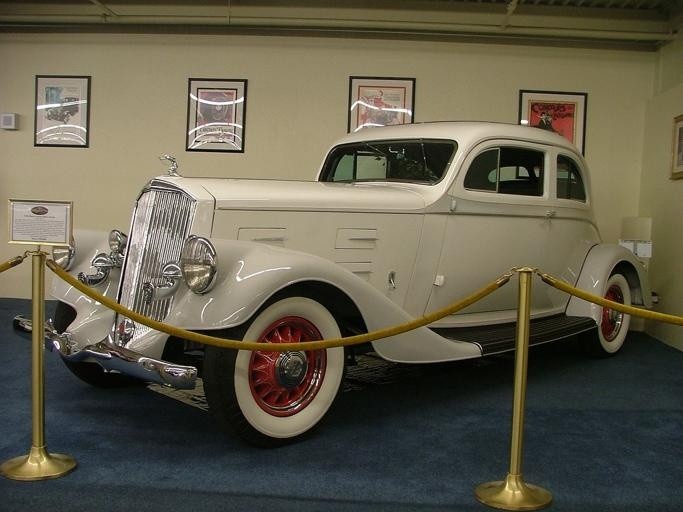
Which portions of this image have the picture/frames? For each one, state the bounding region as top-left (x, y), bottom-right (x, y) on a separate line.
top-left (517, 89), bottom-right (588, 158)
top-left (185, 78), bottom-right (248, 153)
top-left (346, 75), bottom-right (416, 135)
top-left (668, 110), bottom-right (683, 181)
top-left (33, 75), bottom-right (91, 149)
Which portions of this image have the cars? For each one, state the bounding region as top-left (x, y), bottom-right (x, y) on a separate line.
top-left (47, 98), bottom-right (79, 124)
top-left (14, 120), bottom-right (659, 449)
